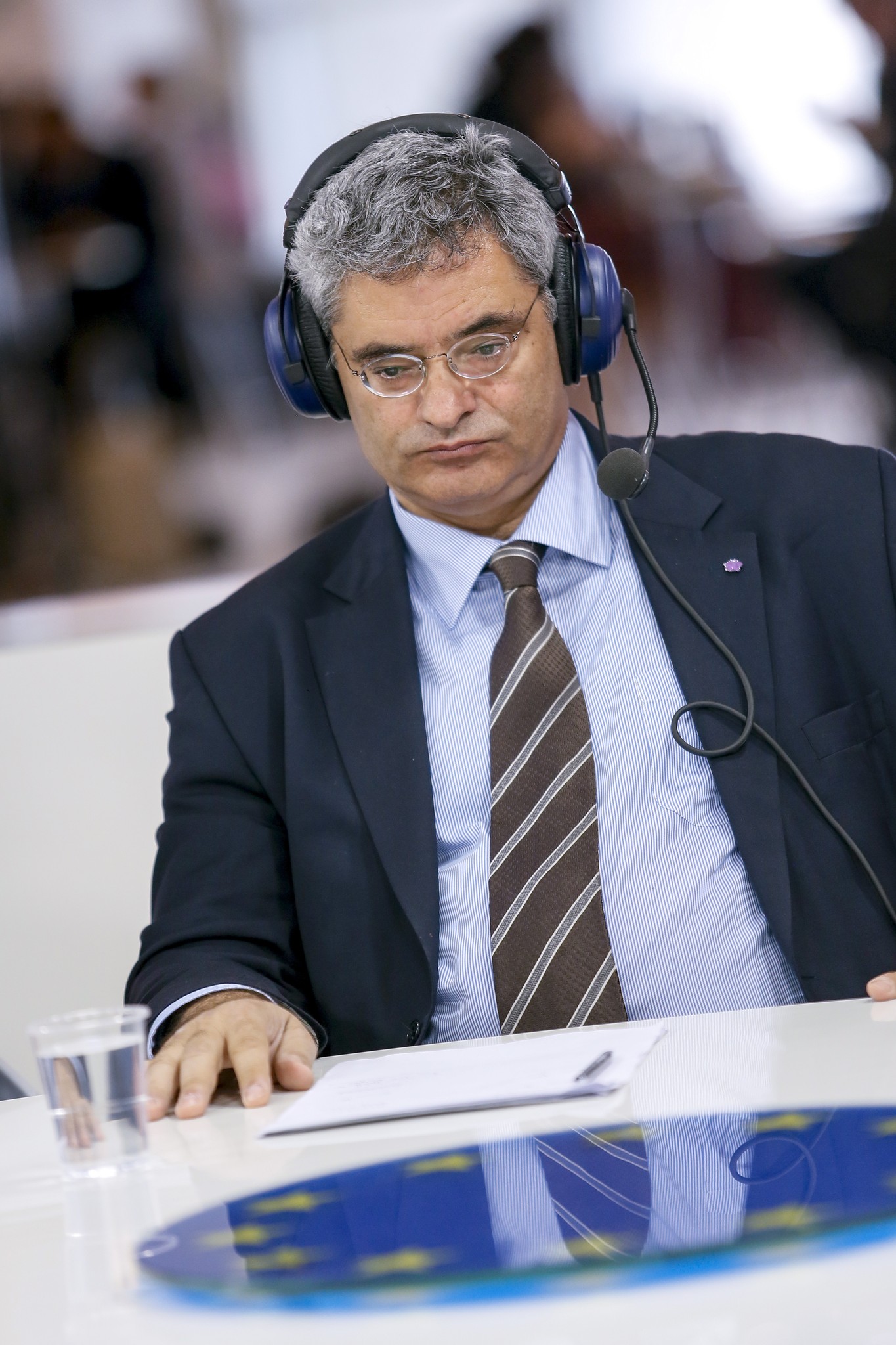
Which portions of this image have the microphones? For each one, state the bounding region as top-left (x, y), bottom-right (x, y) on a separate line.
top-left (598, 290), bottom-right (662, 502)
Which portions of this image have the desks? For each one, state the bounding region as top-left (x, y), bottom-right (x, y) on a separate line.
top-left (0, 996), bottom-right (896, 1344)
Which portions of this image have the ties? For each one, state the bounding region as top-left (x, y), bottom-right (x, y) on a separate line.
top-left (481, 544), bottom-right (634, 1032)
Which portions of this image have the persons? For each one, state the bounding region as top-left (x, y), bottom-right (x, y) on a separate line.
top-left (473, 22), bottom-right (661, 350)
top-left (2, 86), bottom-right (212, 595)
top-left (115, 107), bottom-right (896, 1127)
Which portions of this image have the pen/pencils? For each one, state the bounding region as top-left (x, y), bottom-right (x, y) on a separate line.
top-left (570, 1051), bottom-right (615, 1092)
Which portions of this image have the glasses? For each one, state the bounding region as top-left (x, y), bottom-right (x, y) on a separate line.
top-left (319, 283), bottom-right (545, 398)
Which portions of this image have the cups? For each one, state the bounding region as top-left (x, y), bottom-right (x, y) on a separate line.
top-left (27, 1005), bottom-right (155, 1179)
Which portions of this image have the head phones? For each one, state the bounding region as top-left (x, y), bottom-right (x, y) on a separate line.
top-left (252, 113), bottom-right (660, 428)
